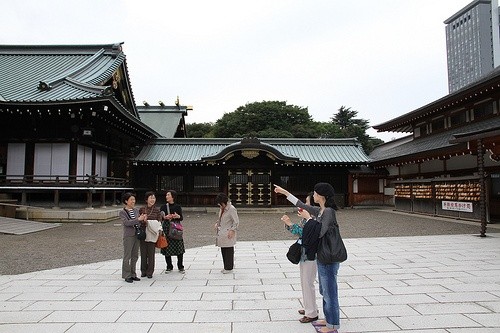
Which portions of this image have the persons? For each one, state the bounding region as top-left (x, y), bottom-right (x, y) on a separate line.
top-left (119, 192), bottom-right (147, 283)
top-left (273, 182), bottom-right (348, 333)
top-left (160, 190), bottom-right (185, 274)
top-left (139, 191), bottom-right (162, 278)
top-left (215, 192), bottom-right (239, 274)
top-left (280, 192), bottom-right (320, 323)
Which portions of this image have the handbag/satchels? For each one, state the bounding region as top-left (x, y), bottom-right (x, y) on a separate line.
top-left (168, 222), bottom-right (184, 240)
top-left (155, 235), bottom-right (168, 248)
top-left (286, 237), bottom-right (303, 264)
top-left (136, 226), bottom-right (146, 240)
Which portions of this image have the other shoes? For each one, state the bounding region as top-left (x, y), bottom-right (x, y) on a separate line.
top-left (125, 278), bottom-right (133, 283)
top-left (298, 310), bottom-right (305, 315)
top-left (311, 319), bottom-right (326, 326)
top-left (178, 268), bottom-right (184, 271)
top-left (141, 274), bottom-right (146, 277)
top-left (315, 326), bottom-right (338, 333)
top-left (166, 267), bottom-right (173, 271)
top-left (148, 275), bottom-right (152, 278)
top-left (131, 276), bottom-right (139, 281)
top-left (223, 269), bottom-right (230, 274)
top-left (299, 316), bottom-right (318, 323)
